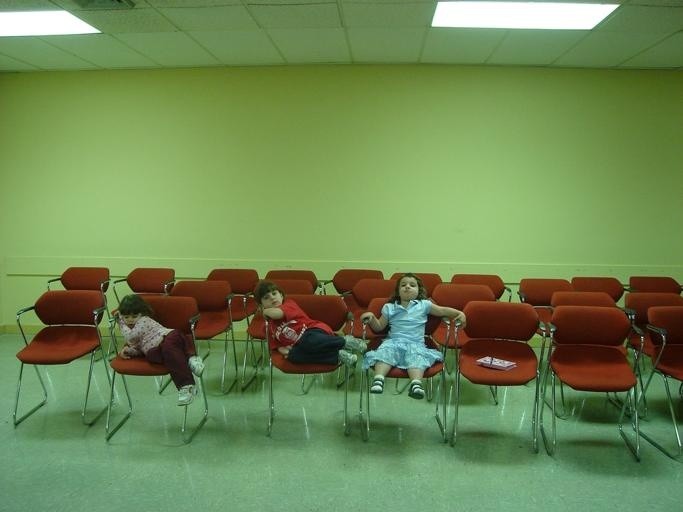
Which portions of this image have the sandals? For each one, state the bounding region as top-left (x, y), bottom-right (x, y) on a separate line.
top-left (408, 382), bottom-right (424, 399)
top-left (370, 378), bottom-right (384, 393)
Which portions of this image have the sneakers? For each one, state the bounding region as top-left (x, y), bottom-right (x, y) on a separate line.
top-left (338, 349), bottom-right (358, 369)
top-left (177, 384), bottom-right (198, 406)
top-left (188, 356), bottom-right (206, 377)
top-left (343, 334), bottom-right (368, 354)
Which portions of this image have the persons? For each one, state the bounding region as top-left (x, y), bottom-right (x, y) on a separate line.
top-left (360, 273), bottom-right (466, 398)
top-left (110, 293), bottom-right (204, 406)
top-left (255, 281), bottom-right (367, 367)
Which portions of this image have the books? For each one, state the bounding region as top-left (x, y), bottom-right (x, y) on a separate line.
top-left (475, 355), bottom-right (516, 372)
